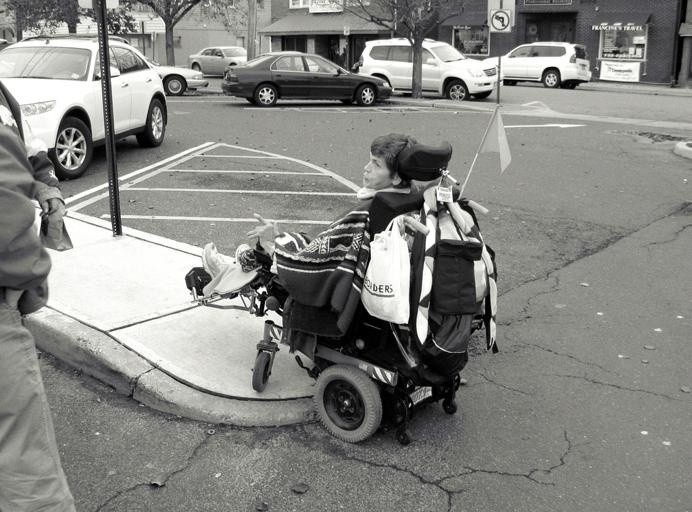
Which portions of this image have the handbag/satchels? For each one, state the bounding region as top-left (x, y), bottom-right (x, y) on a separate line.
top-left (421, 314), bottom-right (471, 379)
top-left (360, 218), bottom-right (414, 325)
top-left (430, 200), bottom-right (484, 316)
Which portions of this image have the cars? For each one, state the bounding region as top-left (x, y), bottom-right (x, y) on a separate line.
top-left (0, 34), bottom-right (209, 180)
top-left (357, 36), bottom-right (504, 101)
top-left (188, 45), bottom-right (247, 76)
top-left (221, 50), bottom-right (394, 107)
top-left (481, 41), bottom-right (593, 89)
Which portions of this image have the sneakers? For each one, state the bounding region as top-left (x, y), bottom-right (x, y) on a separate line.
top-left (201, 243), bottom-right (227, 283)
top-left (234, 242), bottom-right (252, 268)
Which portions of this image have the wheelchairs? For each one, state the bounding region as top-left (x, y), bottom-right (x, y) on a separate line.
top-left (184, 140), bottom-right (460, 448)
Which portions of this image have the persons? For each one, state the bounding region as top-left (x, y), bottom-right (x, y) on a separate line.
top-left (0, 81), bottom-right (76, 511)
top-left (201, 132), bottom-right (419, 309)
